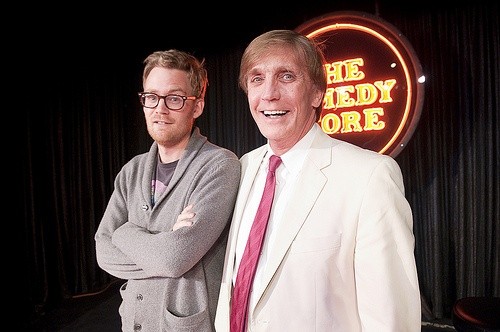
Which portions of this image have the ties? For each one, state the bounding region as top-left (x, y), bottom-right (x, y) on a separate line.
top-left (227, 155), bottom-right (281, 332)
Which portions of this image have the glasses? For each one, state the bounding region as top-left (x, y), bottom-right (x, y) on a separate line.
top-left (137, 91), bottom-right (197, 110)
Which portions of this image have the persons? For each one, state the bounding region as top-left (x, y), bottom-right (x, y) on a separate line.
top-left (94, 50), bottom-right (241, 332)
top-left (215, 29), bottom-right (422, 332)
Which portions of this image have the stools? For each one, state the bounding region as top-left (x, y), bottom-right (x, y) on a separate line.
top-left (452, 296), bottom-right (500, 332)
